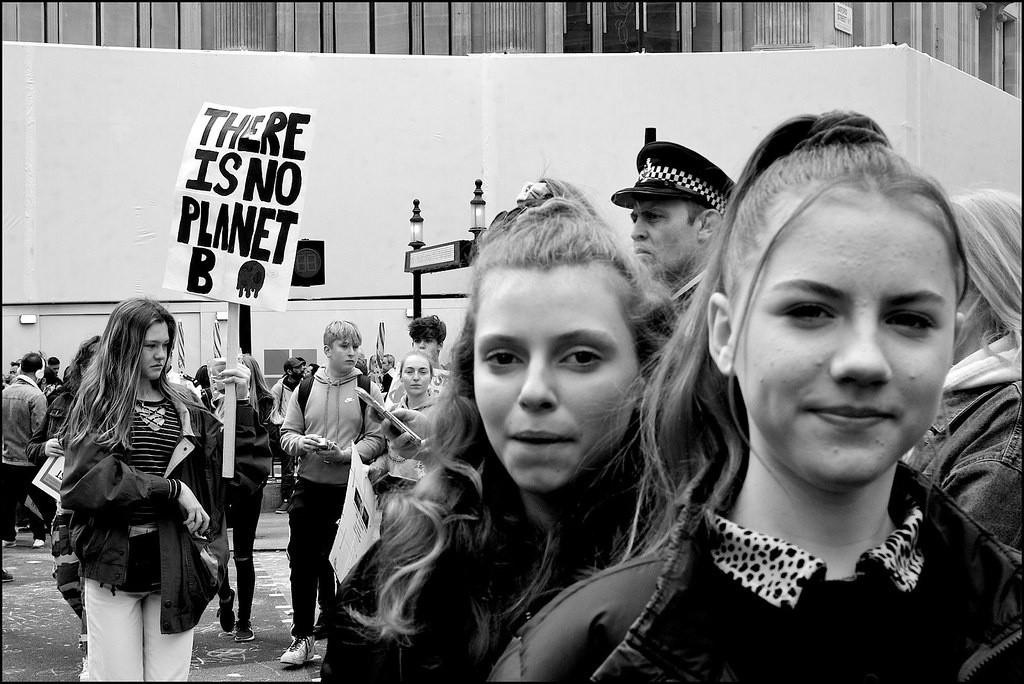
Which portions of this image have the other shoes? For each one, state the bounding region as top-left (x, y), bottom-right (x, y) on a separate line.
top-left (32, 538), bottom-right (44, 549)
top-left (216, 589), bottom-right (235, 632)
top-left (1, 568), bottom-right (14, 582)
top-left (275, 499), bottom-right (290, 514)
top-left (235, 620), bottom-right (254, 642)
top-left (2, 540), bottom-right (17, 548)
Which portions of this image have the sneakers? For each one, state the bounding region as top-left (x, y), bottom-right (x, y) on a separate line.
top-left (280, 634), bottom-right (315, 665)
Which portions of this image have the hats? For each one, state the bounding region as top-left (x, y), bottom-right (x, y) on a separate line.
top-left (284, 358), bottom-right (304, 370)
top-left (611, 141), bottom-right (737, 218)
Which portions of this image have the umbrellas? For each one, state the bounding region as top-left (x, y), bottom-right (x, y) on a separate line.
top-left (376, 321), bottom-right (385, 370)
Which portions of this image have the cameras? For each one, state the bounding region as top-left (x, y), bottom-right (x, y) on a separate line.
top-left (315, 438), bottom-right (329, 450)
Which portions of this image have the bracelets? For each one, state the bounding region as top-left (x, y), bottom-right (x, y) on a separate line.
top-left (169, 478), bottom-right (177, 499)
top-left (174, 478), bottom-right (181, 501)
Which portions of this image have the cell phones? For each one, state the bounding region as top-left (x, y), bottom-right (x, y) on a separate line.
top-left (355, 387), bottom-right (422, 447)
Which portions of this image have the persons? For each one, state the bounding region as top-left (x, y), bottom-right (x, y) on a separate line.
top-left (0, 315), bottom-right (457, 643)
top-left (319, 177), bottom-right (678, 684)
top-left (60, 297), bottom-right (273, 682)
top-left (903, 189), bottom-right (1023, 553)
top-left (278, 319), bottom-right (385, 665)
top-left (609, 141), bottom-right (738, 324)
top-left (26, 336), bottom-right (89, 682)
top-left (482, 109), bottom-right (1024, 684)
top-left (0, 352), bottom-right (55, 547)
top-left (367, 349), bottom-right (440, 506)
